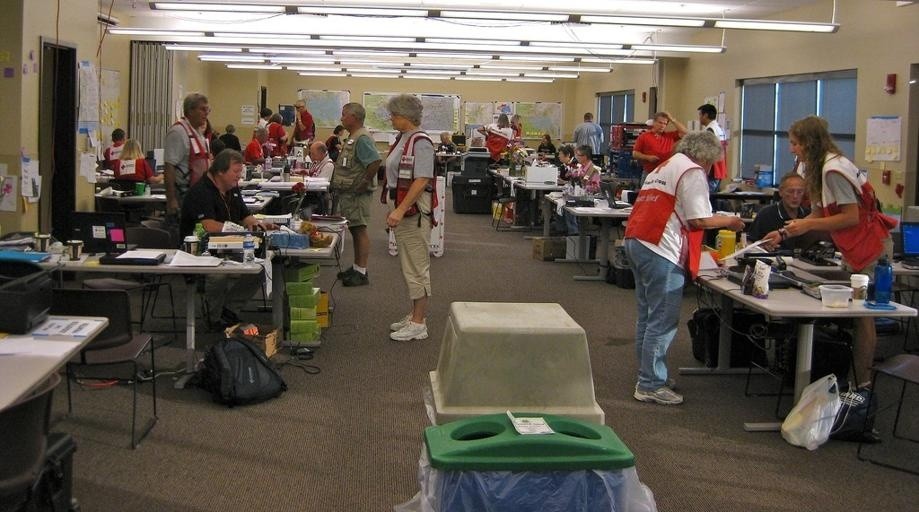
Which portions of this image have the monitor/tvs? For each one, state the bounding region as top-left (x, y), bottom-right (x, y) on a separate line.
top-left (899, 221), bottom-right (919, 265)
top-left (68, 210), bottom-right (128, 254)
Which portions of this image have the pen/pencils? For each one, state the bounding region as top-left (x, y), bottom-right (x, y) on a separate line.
top-left (734, 209), bottom-right (737, 216)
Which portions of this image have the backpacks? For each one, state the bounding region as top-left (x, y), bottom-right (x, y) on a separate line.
top-left (203, 337), bottom-right (288, 408)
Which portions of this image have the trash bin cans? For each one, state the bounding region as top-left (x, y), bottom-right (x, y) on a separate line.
top-left (420, 301), bottom-right (605, 427)
top-left (420, 410), bottom-right (635, 512)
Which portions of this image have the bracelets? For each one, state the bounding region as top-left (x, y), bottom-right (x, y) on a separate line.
top-left (672, 118), bottom-right (676, 123)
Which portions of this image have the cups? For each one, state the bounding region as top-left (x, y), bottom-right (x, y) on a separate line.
top-left (34, 234), bottom-right (51, 253)
top-left (136, 182), bottom-right (146, 195)
top-left (718, 229), bottom-right (737, 260)
top-left (739, 202), bottom-right (754, 218)
top-left (66, 239), bottom-right (84, 261)
top-left (597, 200), bottom-right (608, 210)
top-left (849, 273), bottom-right (869, 306)
top-left (304, 207), bottom-right (312, 222)
top-left (183, 236), bottom-right (200, 256)
top-left (305, 162), bottom-right (312, 169)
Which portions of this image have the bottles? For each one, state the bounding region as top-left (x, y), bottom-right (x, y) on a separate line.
top-left (263, 155), bottom-right (272, 172)
top-left (873, 256), bottom-right (894, 306)
top-left (144, 185), bottom-right (151, 197)
top-left (242, 241), bottom-right (256, 268)
top-left (193, 222), bottom-right (206, 255)
top-left (283, 160), bottom-right (291, 182)
top-left (520, 159), bottom-right (526, 178)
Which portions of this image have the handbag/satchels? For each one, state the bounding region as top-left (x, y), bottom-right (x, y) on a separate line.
top-left (691, 306), bottom-right (767, 367)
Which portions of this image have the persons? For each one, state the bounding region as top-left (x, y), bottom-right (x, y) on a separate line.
top-left (697, 102), bottom-right (728, 192)
top-left (380, 95), bottom-right (439, 341)
top-left (536, 134), bottom-right (601, 195)
top-left (624, 132), bottom-right (745, 405)
top-left (332, 101), bottom-right (382, 286)
top-left (103, 91), bottom-right (342, 331)
top-left (477, 111), bottom-right (522, 164)
top-left (633, 110), bottom-right (688, 187)
top-left (749, 115), bottom-right (899, 391)
top-left (571, 112), bottom-right (604, 166)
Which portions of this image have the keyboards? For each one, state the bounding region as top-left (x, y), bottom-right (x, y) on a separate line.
top-left (904, 265), bottom-right (919, 270)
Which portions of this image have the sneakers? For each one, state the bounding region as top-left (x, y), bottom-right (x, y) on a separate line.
top-left (634, 385), bottom-right (684, 404)
top-left (207, 318), bottom-right (221, 333)
top-left (390, 311), bottom-right (429, 341)
top-left (664, 376), bottom-right (678, 389)
top-left (220, 307), bottom-right (241, 324)
top-left (337, 265), bottom-right (369, 286)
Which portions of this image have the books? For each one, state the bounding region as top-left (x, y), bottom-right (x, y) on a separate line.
top-left (0, 250), bottom-right (50, 262)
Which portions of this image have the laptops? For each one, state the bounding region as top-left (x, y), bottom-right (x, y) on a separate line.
top-left (599, 181), bottom-right (631, 209)
top-left (262, 184), bottom-right (310, 225)
